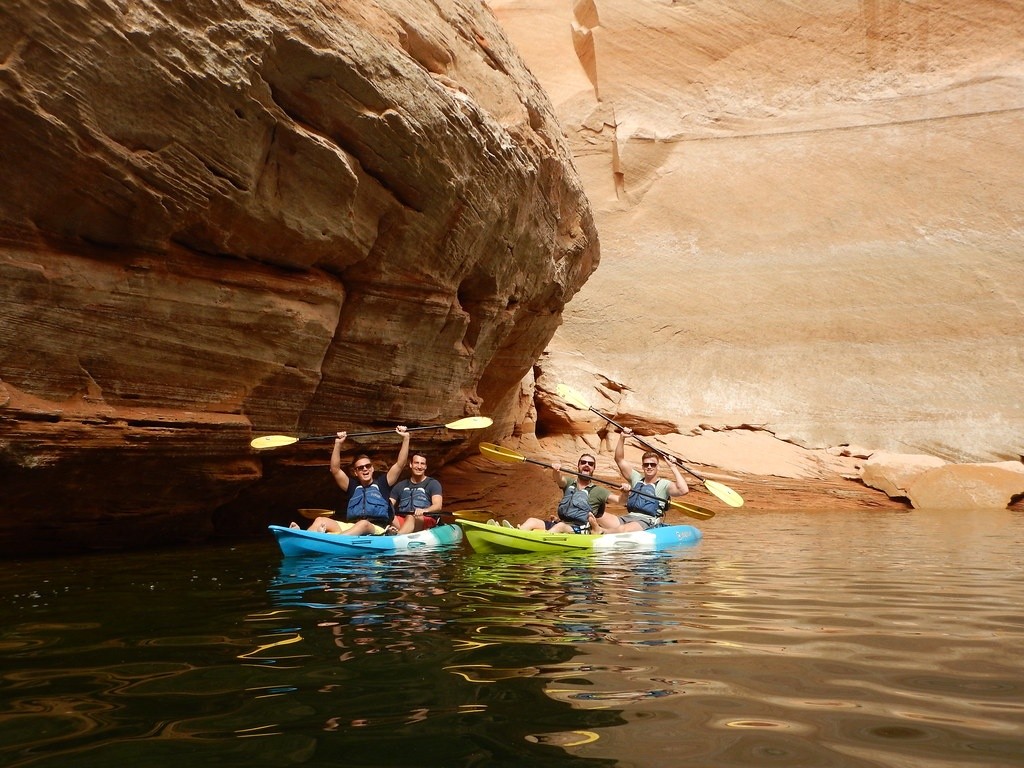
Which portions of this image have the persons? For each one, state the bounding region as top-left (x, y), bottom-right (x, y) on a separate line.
top-left (551, 427), bottom-right (689, 535)
top-left (289, 426), bottom-right (409, 536)
top-left (487, 454), bottom-right (631, 533)
top-left (389, 453), bottom-right (442, 535)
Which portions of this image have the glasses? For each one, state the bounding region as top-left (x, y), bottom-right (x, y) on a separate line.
top-left (356, 463), bottom-right (373, 471)
top-left (580, 460), bottom-right (595, 466)
top-left (643, 463), bottom-right (657, 468)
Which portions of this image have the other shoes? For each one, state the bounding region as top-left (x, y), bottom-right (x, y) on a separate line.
top-left (502, 519), bottom-right (511, 528)
top-left (486, 519), bottom-right (497, 526)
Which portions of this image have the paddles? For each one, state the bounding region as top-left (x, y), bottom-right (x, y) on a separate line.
top-left (479, 442), bottom-right (716, 521)
top-left (555, 384), bottom-right (746, 510)
top-left (250, 417), bottom-right (494, 447)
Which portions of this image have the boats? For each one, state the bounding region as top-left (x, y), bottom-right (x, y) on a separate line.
top-left (454, 516), bottom-right (702, 558)
top-left (267, 521), bottom-right (463, 559)
